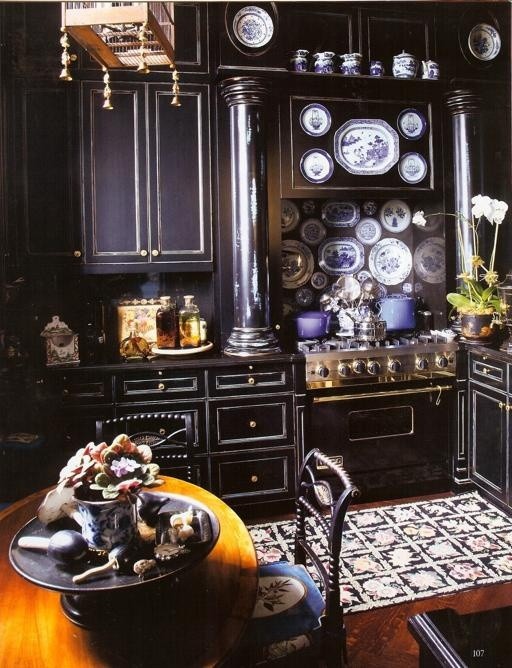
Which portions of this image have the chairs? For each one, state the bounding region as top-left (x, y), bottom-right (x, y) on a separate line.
top-left (96, 413), bottom-right (194, 484)
top-left (243, 449), bottom-right (360, 667)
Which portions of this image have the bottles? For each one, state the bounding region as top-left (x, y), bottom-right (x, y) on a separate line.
top-left (116, 320), bottom-right (152, 360)
top-left (82, 293), bottom-right (113, 364)
top-left (286, 48), bottom-right (386, 75)
top-left (178, 294), bottom-right (202, 348)
top-left (155, 295), bottom-right (178, 350)
top-left (198, 316), bottom-right (207, 343)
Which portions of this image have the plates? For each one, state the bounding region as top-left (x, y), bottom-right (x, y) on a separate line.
top-left (297, 101), bottom-right (430, 185)
top-left (279, 200), bottom-right (414, 306)
top-left (414, 202), bottom-right (447, 293)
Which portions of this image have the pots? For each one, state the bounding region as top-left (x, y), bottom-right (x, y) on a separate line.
top-left (376, 293), bottom-right (421, 334)
top-left (286, 307), bottom-right (335, 338)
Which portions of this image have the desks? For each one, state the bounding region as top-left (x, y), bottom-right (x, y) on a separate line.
top-left (0, 471), bottom-right (258, 668)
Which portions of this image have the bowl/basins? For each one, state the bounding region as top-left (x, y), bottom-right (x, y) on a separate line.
top-left (232, 5), bottom-right (273, 48)
top-left (466, 25), bottom-right (502, 63)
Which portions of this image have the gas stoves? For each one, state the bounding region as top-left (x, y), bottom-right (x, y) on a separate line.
top-left (297, 336), bottom-right (462, 390)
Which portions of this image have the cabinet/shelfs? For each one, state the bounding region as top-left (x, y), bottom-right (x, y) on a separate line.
top-left (208, 355), bottom-right (297, 515)
top-left (0, 1), bottom-right (83, 276)
top-left (112, 364), bottom-right (207, 486)
top-left (465, 346), bottom-right (511, 516)
top-left (0, 367), bottom-right (111, 502)
top-left (78, 2), bottom-right (214, 274)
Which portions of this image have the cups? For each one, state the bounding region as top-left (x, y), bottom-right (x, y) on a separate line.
top-left (496, 271), bottom-right (512, 353)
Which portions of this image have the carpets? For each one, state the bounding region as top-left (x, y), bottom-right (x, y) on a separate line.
top-left (246, 489), bottom-right (512, 615)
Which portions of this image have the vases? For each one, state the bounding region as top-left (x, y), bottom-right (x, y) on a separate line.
top-left (461, 315), bottom-right (495, 345)
top-left (71, 480), bottom-right (141, 552)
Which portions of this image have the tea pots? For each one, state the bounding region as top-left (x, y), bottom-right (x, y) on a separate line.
top-left (421, 56), bottom-right (443, 80)
top-left (393, 48), bottom-right (419, 78)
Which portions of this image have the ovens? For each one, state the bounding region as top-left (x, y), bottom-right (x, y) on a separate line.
top-left (303, 381), bottom-right (451, 478)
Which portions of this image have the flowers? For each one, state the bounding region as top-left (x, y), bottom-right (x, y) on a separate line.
top-left (57, 433), bottom-right (166, 504)
top-left (412, 194), bottom-right (510, 315)
